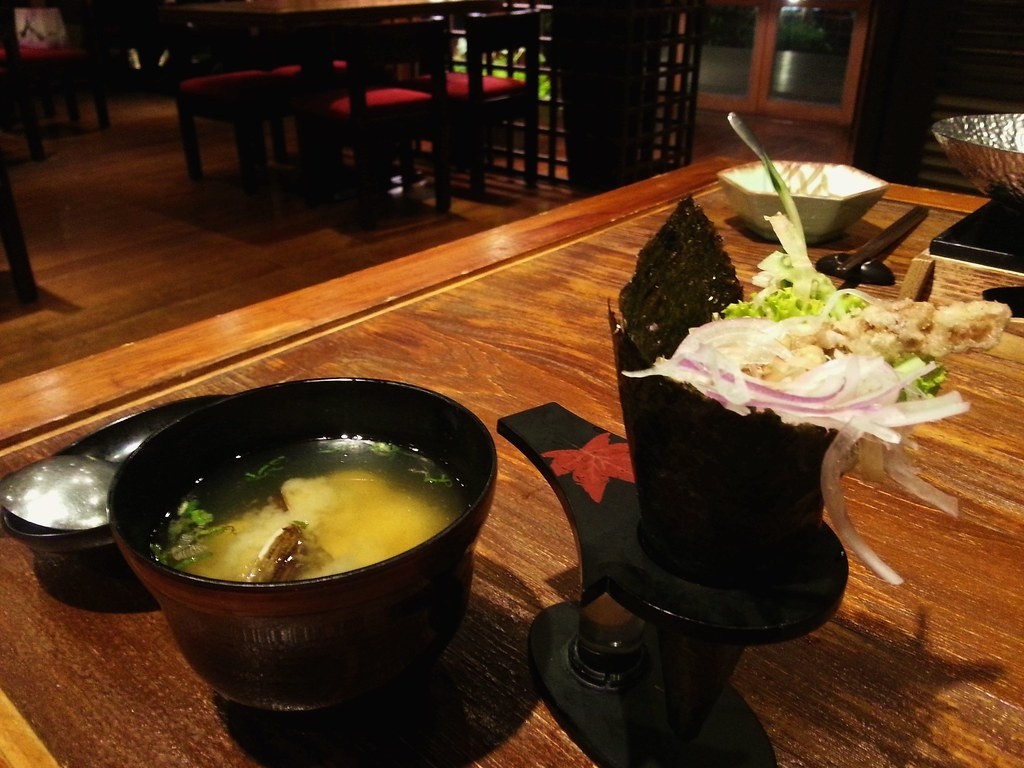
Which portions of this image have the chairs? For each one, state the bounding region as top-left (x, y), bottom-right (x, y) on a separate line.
top-left (0, 0), bottom-right (112, 160)
top-left (165, 8), bottom-right (542, 215)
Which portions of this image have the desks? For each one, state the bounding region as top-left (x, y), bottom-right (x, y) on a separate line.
top-left (163, 0), bottom-right (502, 208)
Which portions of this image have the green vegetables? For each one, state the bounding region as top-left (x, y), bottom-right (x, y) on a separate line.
top-left (148, 441), bottom-right (453, 568)
top-left (721, 281), bottom-right (950, 402)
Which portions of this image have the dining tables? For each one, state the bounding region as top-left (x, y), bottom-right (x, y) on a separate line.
top-left (0, 155), bottom-right (1024, 767)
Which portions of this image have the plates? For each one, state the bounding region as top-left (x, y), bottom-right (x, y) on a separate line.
top-left (0, 393), bottom-right (234, 549)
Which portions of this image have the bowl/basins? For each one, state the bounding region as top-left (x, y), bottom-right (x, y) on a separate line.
top-left (118, 378), bottom-right (499, 709)
top-left (715, 158), bottom-right (889, 245)
top-left (930, 111), bottom-right (1024, 202)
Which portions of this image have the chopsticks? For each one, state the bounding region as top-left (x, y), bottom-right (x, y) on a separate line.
top-left (836, 202), bottom-right (930, 273)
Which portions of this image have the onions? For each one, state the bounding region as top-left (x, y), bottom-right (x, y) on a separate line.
top-left (623, 317), bottom-right (970, 586)
